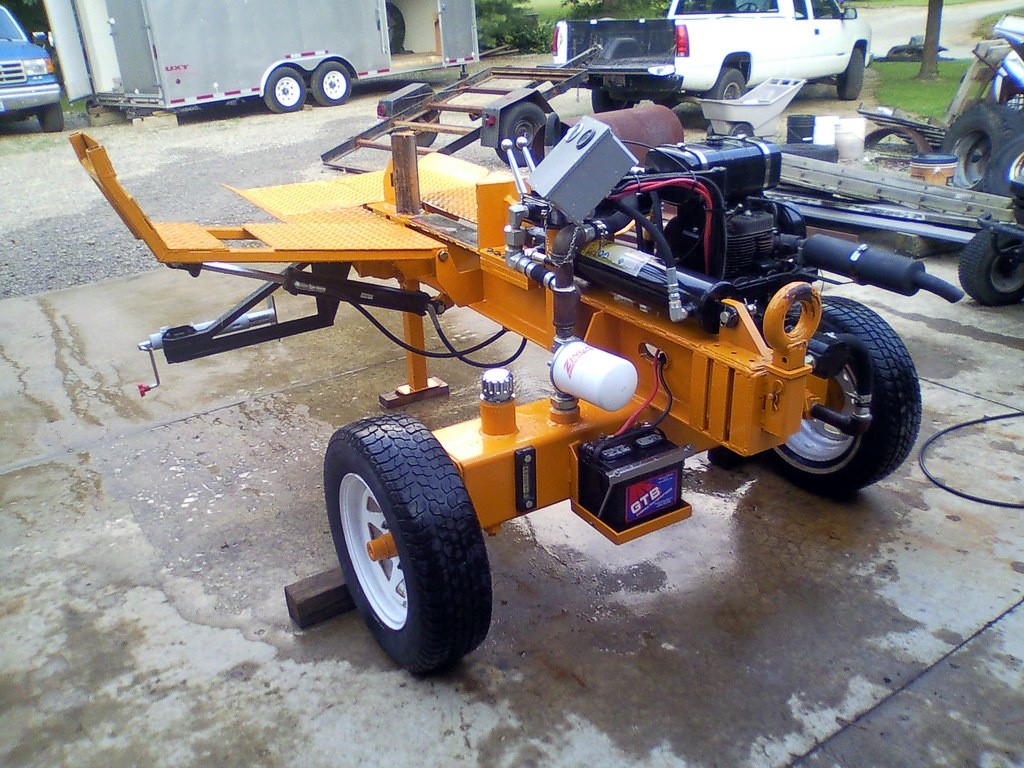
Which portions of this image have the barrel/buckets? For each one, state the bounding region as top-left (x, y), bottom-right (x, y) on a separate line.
top-left (836, 118), bottom-right (865, 161)
top-left (786, 115), bottom-right (815, 144)
top-left (910, 153), bottom-right (958, 186)
top-left (812, 116), bottom-right (839, 145)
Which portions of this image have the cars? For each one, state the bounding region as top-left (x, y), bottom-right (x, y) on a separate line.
top-left (0, 5), bottom-right (64, 132)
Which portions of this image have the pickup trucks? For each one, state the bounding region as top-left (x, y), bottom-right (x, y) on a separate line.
top-left (553, 0), bottom-right (872, 113)
